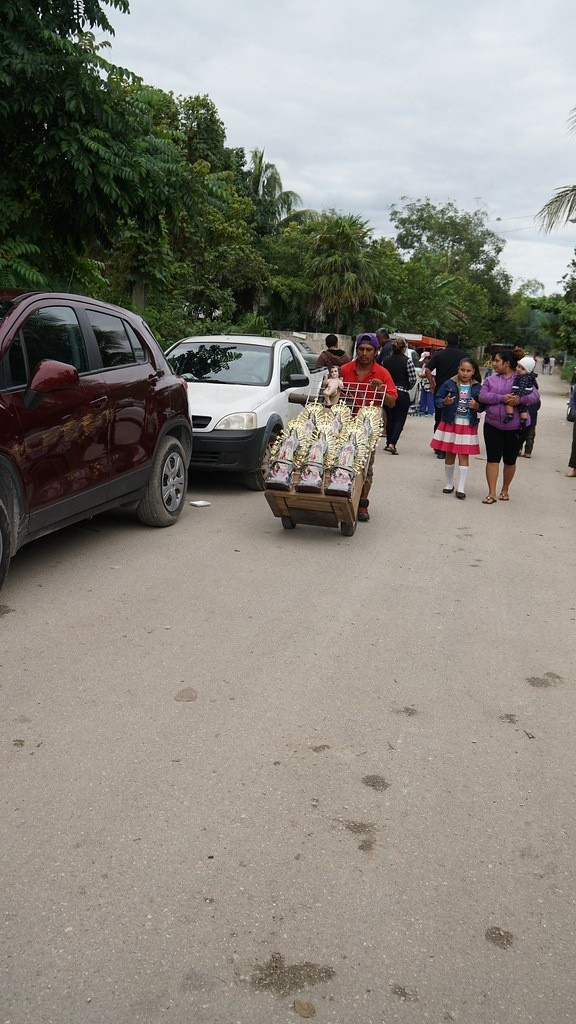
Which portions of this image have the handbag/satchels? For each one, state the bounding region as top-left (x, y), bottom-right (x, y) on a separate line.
top-left (567, 410), bottom-right (576, 422)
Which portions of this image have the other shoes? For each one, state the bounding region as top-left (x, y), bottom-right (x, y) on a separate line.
top-left (519, 420), bottom-right (526, 431)
top-left (385, 444), bottom-right (398, 455)
top-left (566, 471), bottom-right (576, 477)
top-left (443, 486), bottom-right (454, 493)
top-left (524, 452), bottom-right (531, 458)
top-left (435, 450), bottom-right (446, 459)
top-left (456, 491), bottom-right (466, 498)
top-left (503, 414), bottom-right (513, 423)
top-left (415, 412), bottom-right (433, 417)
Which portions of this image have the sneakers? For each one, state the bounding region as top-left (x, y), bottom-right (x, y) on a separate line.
top-left (358, 498), bottom-right (370, 521)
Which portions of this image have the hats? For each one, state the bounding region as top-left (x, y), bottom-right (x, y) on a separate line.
top-left (357, 333), bottom-right (379, 349)
top-left (419, 352), bottom-right (430, 362)
top-left (517, 356), bottom-right (536, 374)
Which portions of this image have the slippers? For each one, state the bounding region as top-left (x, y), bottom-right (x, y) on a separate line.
top-left (482, 495), bottom-right (497, 504)
top-left (499, 493), bottom-right (509, 500)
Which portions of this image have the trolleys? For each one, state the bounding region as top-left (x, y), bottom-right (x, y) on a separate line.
top-left (263, 380), bottom-right (386, 536)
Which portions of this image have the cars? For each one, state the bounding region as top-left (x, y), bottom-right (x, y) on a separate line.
top-left (405, 349), bottom-right (422, 405)
top-left (163, 335), bottom-right (311, 490)
top-left (0, 289), bottom-right (193, 585)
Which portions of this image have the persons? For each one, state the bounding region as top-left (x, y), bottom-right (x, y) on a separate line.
top-left (300, 431), bottom-right (328, 463)
top-left (543, 354), bottom-right (550, 375)
top-left (380, 337), bottom-right (417, 454)
top-left (503, 356), bottom-right (535, 431)
top-left (336, 434), bottom-right (359, 468)
top-left (330, 412), bottom-right (344, 434)
top-left (374, 328), bottom-right (393, 365)
top-left (318, 334), bottom-right (350, 378)
top-left (565, 367), bottom-right (576, 477)
top-left (321, 365), bottom-right (344, 407)
top-left (483, 355), bottom-right (494, 378)
top-left (416, 351), bottom-right (436, 418)
top-left (549, 356), bottom-right (555, 375)
top-left (303, 413), bottom-right (317, 433)
top-left (338, 334), bottom-right (398, 521)
top-left (434, 358), bottom-right (486, 499)
top-left (274, 427), bottom-right (299, 462)
top-left (359, 416), bottom-right (373, 444)
top-left (478, 349), bottom-right (540, 504)
top-left (425, 331), bottom-right (483, 459)
top-left (513, 347), bottom-right (541, 458)
